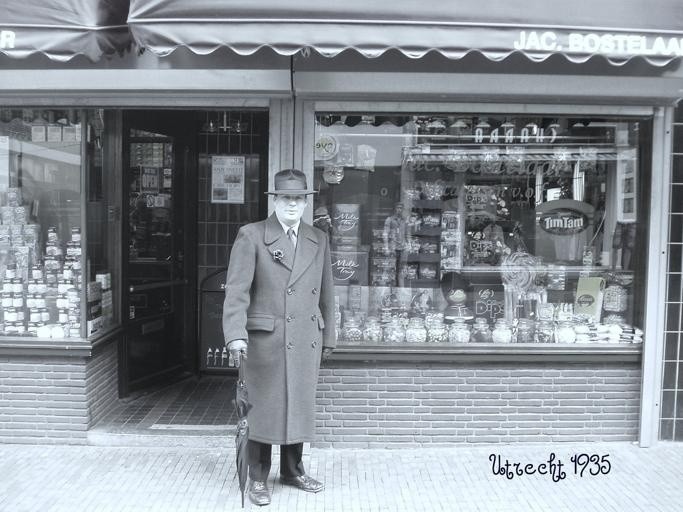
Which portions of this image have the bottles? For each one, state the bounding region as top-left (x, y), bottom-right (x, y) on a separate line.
top-left (1, 170), bottom-right (81, 338)
top-left (330, 279), bottom-right (643, 344)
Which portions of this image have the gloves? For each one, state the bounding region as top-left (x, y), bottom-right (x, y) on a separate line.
top-left (224, 339), bottom-right (250, 370)
top-left (320, 345), bottom-right (332, 364)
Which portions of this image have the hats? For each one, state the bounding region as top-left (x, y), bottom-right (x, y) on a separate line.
top-left (393, 201), bottom-right (403, 208)
top-left (262, 167), bottom-right (320, 198)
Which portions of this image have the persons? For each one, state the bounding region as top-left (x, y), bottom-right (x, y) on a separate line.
top-left (383, 202), bottom-right (410, 287)
top-left (231, 355), bottom-right (253, 508)
top-left (222, 169), bottom-right (336, 506)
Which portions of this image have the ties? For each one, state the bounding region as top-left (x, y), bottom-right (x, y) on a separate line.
top-left (286, 227), bottom-right (296, 248)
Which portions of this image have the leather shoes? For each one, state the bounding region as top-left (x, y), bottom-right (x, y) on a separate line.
top-left (247, 477), bottom-right (272, 508)
top-left (279, 473), bottom-right (325, 494)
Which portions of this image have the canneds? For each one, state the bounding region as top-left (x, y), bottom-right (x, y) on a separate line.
top-left (0, 187), bottom-right (85, 340)
top-left (334, 284), bottom-right (576, 348)
top-left (31, 123), bottom-right (91, 143)
top-left (442, 210), bottom-right (463, 272)
top-left (582, 245), bottom-right (594, 266)
